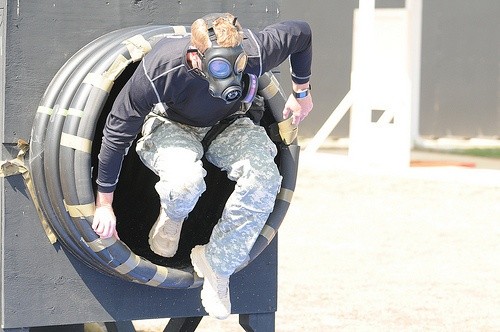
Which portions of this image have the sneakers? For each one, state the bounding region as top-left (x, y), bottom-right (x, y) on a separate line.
top-left (149, 205), bottom-right (182, 258)
top-left (190, 245), bottom-right (231, 320)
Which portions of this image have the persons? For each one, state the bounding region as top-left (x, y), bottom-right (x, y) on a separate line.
top-left (91, 12), bottom-right (313, 320)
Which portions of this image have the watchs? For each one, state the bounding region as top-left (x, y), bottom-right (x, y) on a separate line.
top-left (292, 83), bottom-right (312, 98)
